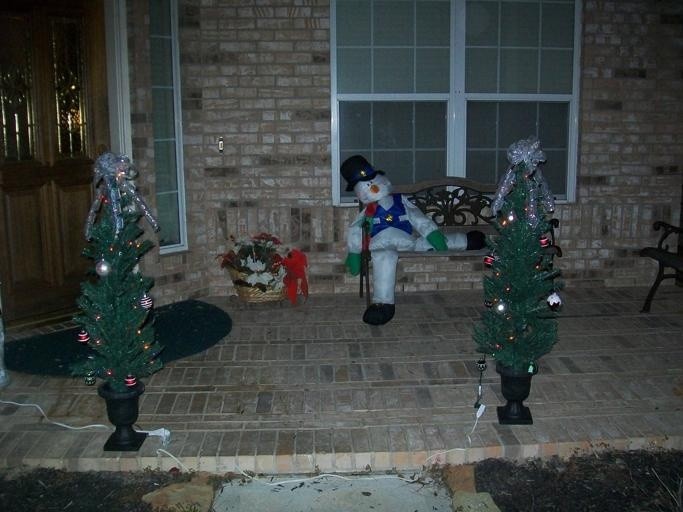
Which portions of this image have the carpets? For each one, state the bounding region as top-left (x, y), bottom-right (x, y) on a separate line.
top-left (5, 299), bottom-right (232, 378)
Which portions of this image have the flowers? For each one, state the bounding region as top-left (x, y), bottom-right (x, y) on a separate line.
top-left (214, 232), bottom-right (308, 305)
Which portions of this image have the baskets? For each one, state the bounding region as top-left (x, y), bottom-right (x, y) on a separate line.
top-left (227, 265), bottom-right (287, 302)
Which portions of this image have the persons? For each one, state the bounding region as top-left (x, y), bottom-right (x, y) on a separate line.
top-left (340, 154), bottom-right (488, 327)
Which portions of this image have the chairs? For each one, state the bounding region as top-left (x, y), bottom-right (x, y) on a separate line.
top-left (640, 222), bottom-right (683, 312)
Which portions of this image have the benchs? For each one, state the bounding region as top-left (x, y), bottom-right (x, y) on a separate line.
top-left (360, 177), bottom-right (562, 308)
